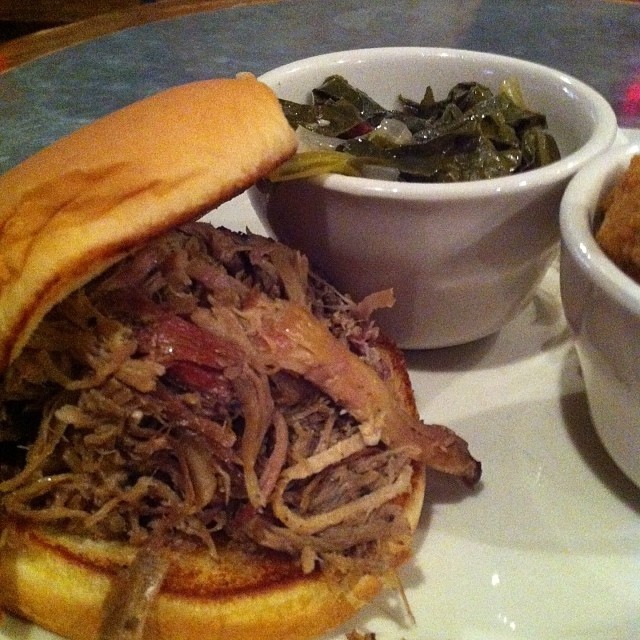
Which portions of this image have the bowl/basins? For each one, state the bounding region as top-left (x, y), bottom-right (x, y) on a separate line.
top-left (557, 140), bottom-right (640, 493)
top-left (246, 44), bottom-right (619, 353)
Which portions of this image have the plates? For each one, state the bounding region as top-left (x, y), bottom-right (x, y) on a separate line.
top-left (3, 124), bottom-right (638, 640)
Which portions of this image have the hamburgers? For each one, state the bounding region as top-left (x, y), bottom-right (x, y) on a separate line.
top-left (0, 73), bottom-right (481, 640)
